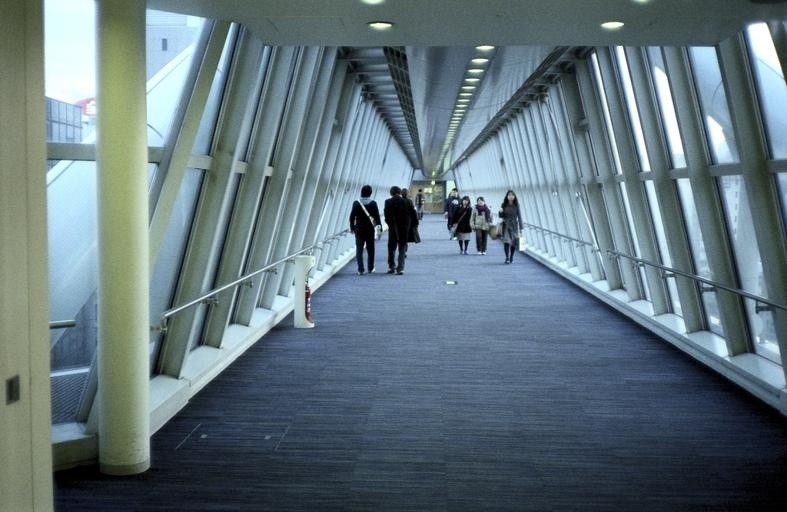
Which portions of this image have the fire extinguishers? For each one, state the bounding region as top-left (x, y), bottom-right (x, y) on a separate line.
top-left (305, 282), bottom-right (311, 321)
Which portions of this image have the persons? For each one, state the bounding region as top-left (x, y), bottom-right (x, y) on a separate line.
top-left (451, 197), bottom-right (472, 255)
top-left (384, 187), bottom-right (421, 275)
top-left (415, 189), bottom-right (426, 220)
top-left (499, 190), bottom-right (523, 264)
top-left (401, 189), bottom-right (413, 257)
top-left (445, 189), bottom-right (462, 240)
top-left (350, 186), bottom-right (382, 275)
top-left (469, 197), bottom-right (492, 255)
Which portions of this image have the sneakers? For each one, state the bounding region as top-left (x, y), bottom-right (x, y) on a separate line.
top-left (464, 250), bottom-right (470, 255)
top-left (396, 269), bottom-right (404, 275)
top-left (510, 258), bottom-right (515, 264)
top-left (368, 267), bottom-right (375, 274)
top-left (358, 271), bottom-right (365, 276)
top-left (477, 251), bottom-right (482, 255)
top-left (481, 251), bottom-right (486, 255)
top-left (505, 257), bottom-right (510, 264)
top-left (460, 250), bottom-right (464, 255)
top-left (385, 268), bottom-right (395, 274)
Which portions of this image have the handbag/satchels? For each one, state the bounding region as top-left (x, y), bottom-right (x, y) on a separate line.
top-left (448, 223), bottom-right (458, 237)
top-left (373, 225), bottom-right (383, 241)
top-left (515, 236), bottom-right (526, 252)
top-left (488, 225), bottom-right (498, 240)
top-left (495, 221), bottom-right (506, 238)
